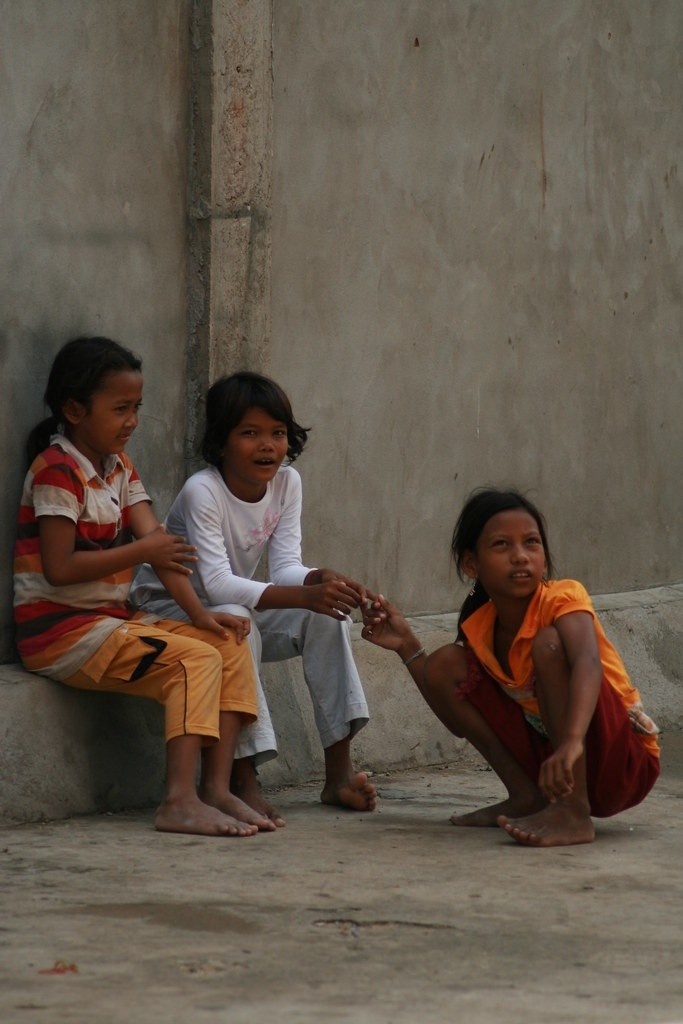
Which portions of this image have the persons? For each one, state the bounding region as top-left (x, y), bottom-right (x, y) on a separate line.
top-left (13, 335), bottom-right (276, 836)
top-left (130, 372), bottom-right (376, 826)
top-left (361, 489), bottom-right (661, 847)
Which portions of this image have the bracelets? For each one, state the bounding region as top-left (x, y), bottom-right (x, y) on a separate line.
top-left (405, 648), bottom-right (425, 666)
